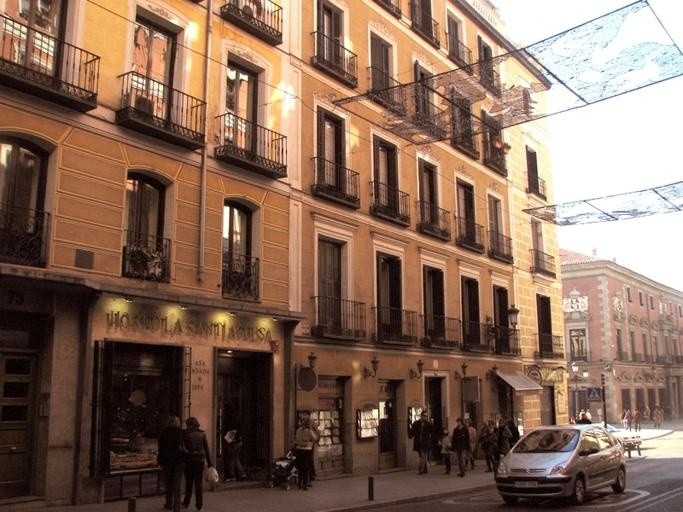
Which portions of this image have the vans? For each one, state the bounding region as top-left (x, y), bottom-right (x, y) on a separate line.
top-left (494, 423), bottom-right (626, 508)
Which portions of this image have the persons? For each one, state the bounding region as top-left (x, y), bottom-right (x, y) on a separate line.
top-left (286, 409), bottom-right (321, 490)
top-left (570, 402), bottom-right (665, 431)
top-left (157, 416), bottom-right (213, 512)
top-left (223, 423), bottom-right (249, 482)
top-left (411, 410), bottom-right (521, 478)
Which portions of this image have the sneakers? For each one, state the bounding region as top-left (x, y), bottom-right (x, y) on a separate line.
top-left (164, 500), bottom-right (202, 512)
top-left (298, 484), bottom-right (309, 490)
top-left (457, 472), bottom-right (465, 477)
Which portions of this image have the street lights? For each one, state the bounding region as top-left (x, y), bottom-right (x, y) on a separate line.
top-left (570, 362), bottom-right (581, 423)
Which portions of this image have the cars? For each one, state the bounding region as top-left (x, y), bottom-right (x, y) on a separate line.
top-left (599, 421), bottom-right (642, 449)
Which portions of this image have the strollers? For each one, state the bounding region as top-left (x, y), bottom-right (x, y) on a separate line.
top-left (261, 448), bottom-right (295, 490)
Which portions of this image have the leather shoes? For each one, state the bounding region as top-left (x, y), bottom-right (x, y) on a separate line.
top-left (417, 468), bottom-right (429, 475)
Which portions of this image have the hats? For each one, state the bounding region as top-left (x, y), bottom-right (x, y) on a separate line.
top-left (498, 418), bottom-right (513, 424)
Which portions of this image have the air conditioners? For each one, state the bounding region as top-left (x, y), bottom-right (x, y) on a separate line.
top-left (233, 0), bottom-right (259, 24)
top-left (122, 245), bottom-right (165, 280)
top-left (130, 90), bottom-right (157, 116)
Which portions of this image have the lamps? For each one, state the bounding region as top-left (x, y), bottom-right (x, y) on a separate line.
top-left (454, 363), bottom-right (468, 380)
top-left (486, 305), bottom-right (519, 338)
top-left (300, 351), bottom-right (317, 371)
top-left (486, 364), bottom-right (498, 382)
top-left (409, 359), bottom-right (424, 379)
top-left (364, 356), bottom-right (379, 378)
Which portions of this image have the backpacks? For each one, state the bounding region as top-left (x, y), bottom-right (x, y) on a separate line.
top-left (503, 420), bottom-right (520, 443)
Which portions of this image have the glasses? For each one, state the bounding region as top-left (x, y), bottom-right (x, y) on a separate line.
top-left (423, 414), bottom-right (428, 416)
